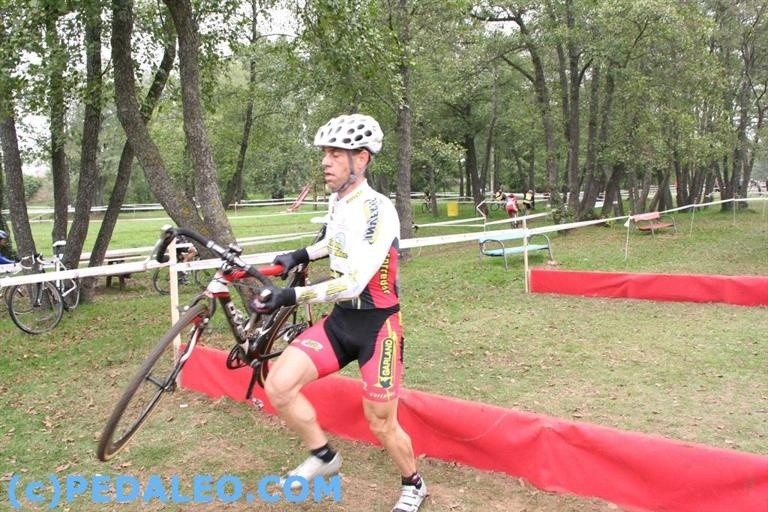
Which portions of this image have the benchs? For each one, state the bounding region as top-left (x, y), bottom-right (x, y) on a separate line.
top-left (633, 211), bottom-right (677, 238)
top-left (478, 228), bottom-right (554, 270)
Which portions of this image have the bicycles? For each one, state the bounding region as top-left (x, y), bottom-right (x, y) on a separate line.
top-left (8, 240), bottom-right (81, 335)
top-left (0, 253), bottom-right (35, 315)
top-left (490, 197), bottom-right (507, 212)
top-left (153, 252), bottom-right (212, 297)
top-left (421, 197), bottom-right (432, 214)
top-left (523, 205), bottom-right (537, 223)
top-left (510, 209), bottom-right (522, 230)
top-left (96, 211), bottom-right (329, 463)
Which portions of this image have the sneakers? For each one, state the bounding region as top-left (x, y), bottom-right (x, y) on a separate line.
top-left (275, 451), bottom-right (343, 490)
top-left (391, 477), bottom-right (427, 511)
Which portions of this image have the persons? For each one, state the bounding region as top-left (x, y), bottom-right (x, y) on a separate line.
top-left (504, 194), bottom-right (521, 226)
top-left (152, 224), bottom-right (199, 283)
top-left (523, 189), bottom-right (535, 217)
top-left (0, 228), bottom-right (19, 267)
top-left (495, 188), bottom-right (506, 209)
top-left (247, 111), bottom-right (429, 511)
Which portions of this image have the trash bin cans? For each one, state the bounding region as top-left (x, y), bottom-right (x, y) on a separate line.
top-left (447, 201), bottom-right (459, 218)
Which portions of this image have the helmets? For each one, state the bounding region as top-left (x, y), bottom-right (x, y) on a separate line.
top-left (0, 231), bottom-right (9, 239)
top-left (314, 113), bottom-right (384, 155)
